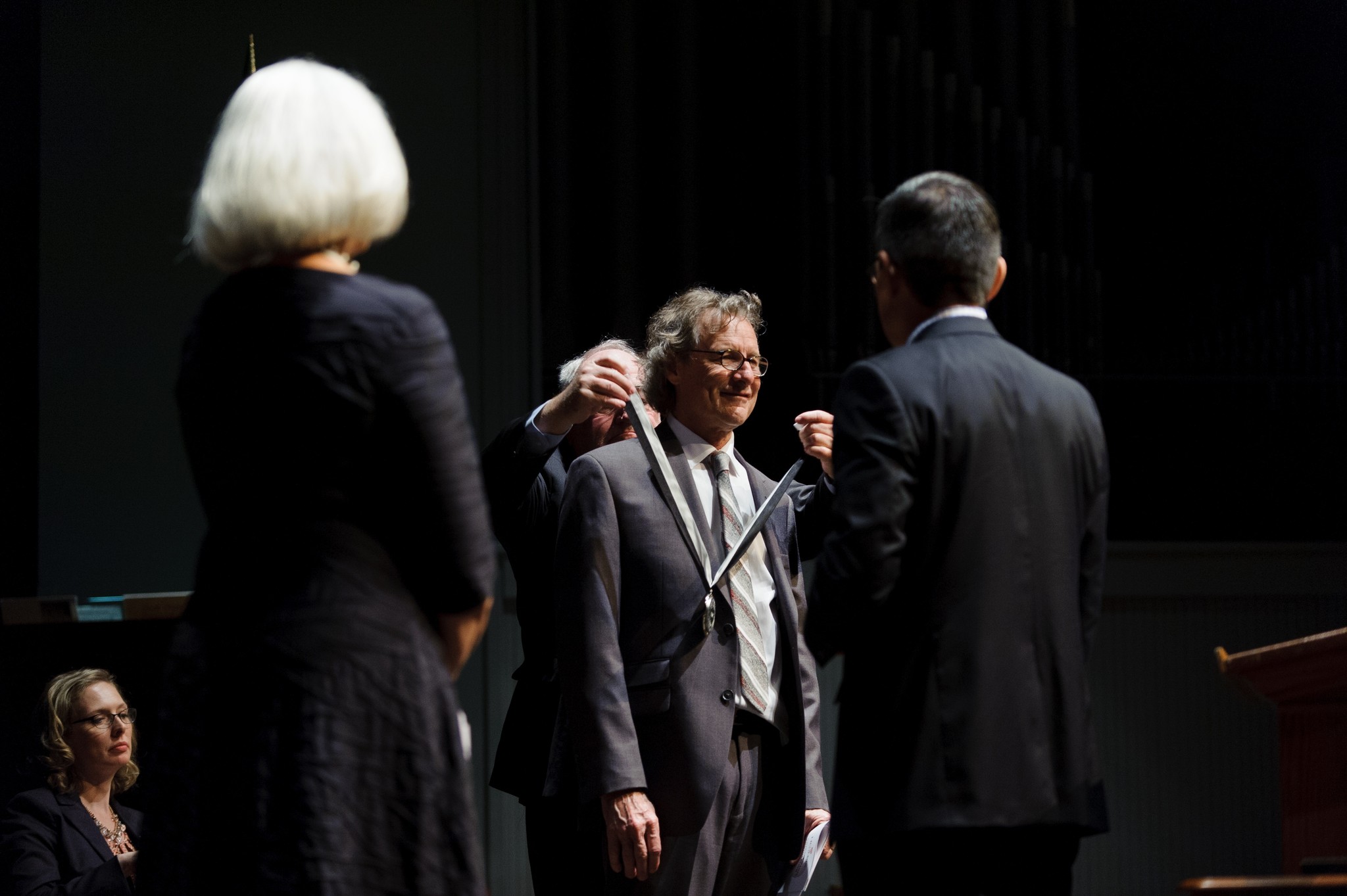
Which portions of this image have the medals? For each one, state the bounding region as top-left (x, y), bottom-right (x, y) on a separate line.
top-left (703, 593), bottom-right (716, 637)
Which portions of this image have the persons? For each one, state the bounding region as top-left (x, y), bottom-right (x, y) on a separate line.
top-left (804, 173), bottom-right (1119, 896)
top-left (0, 669), bottom-right (157, 896)
top-left (478, 285), bottom-right (834, 896)
top-left (176, 58), bottom-right (496, 896)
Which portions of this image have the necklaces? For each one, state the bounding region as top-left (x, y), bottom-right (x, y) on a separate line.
top-left (86, 803), bottom-right (126, 846)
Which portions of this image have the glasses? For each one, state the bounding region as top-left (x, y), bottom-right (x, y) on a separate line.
top-left (675, 347), bottom-right (769, 377)
top-left (70, 707), bottom-right (137, 729)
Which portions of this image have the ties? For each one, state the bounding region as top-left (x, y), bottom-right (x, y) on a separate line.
top-left (707, 450), bottom-right (770, 715)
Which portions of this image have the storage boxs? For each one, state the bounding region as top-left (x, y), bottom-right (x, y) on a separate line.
top-left (123, 592), bottom-right (192, 621)
